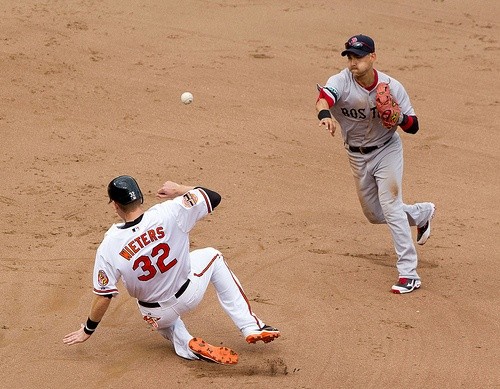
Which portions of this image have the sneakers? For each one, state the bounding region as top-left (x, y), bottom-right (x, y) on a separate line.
top-left (391, 278), bottom-right (421, 294)
top-left (242, 324), bottom-right (280, 344)
top-left (417, 202), bottom-right (436, 245)
top-left (188, 337), bottom-right (238, 365)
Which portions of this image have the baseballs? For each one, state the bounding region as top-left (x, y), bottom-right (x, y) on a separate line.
top-left (181, 92), bottom-right (194, 105)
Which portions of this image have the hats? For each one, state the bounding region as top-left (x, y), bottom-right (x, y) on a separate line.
top-left (341, 34), bottom-right (375, 57)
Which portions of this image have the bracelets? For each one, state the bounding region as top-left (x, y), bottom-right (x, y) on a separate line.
top-left (83, 316), bottom-right (99, 335)
top-left (317, 109), bottom-right (333, 121)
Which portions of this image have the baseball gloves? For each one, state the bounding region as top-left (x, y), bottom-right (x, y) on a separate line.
top-left (376, 83), bottom-right (401, 129)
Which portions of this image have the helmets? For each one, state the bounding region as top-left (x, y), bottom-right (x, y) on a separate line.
top-left (108, 175), bottom-right (143, 204)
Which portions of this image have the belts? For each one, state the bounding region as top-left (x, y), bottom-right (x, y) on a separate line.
top-left (138, 279), bottom-right (191, 308)
top-left (349, 137), bottom-right (392, 154)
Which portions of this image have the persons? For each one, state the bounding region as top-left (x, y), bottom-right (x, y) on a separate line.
top-left (315, 36), bottom-right (435, 295)
top-left (62, 176), bottom-right (281, 367)
